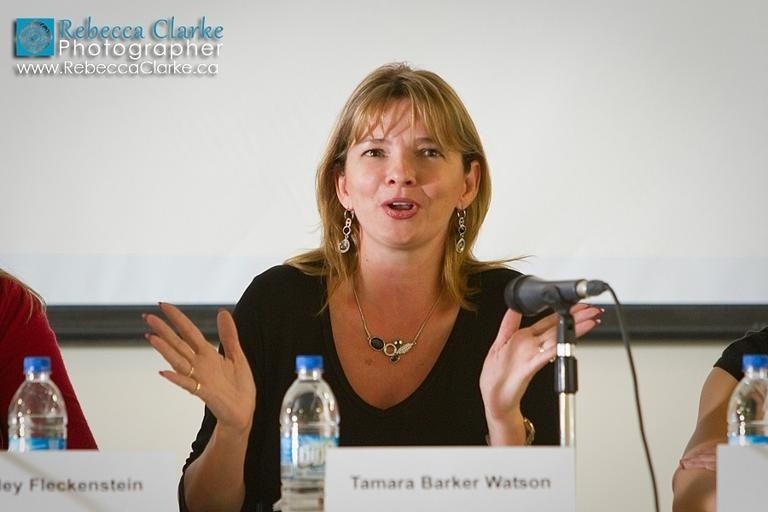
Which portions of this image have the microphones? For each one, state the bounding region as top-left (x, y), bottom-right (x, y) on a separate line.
top-left (504, 275), bottom-right (607, 317)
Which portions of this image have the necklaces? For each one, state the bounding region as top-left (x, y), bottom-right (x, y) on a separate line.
top-left (346, 270), bottom-right (447, 364)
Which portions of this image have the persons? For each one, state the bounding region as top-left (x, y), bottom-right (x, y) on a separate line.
top-left (138, 59), bottom-right (605, 512)
top-left (670, 322), bottom-right (767, 512)
top-left (0, 269), bottom-right (99, 449)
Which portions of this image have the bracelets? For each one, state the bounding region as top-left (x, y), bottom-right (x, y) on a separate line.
top-left (482, 415), bottom-right (535, 445)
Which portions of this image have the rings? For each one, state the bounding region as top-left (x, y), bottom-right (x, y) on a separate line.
top-left (191, 381), bottom-right (200, 396)
top-left (538, 345), bottom-right (545, 353)
top-left (187, 367), bottom-right (194, 376)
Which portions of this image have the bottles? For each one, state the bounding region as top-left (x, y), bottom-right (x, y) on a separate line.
top-left (726, 353), bottom-right (768, 447)
top-left (280, 355), bottom-right (341, 512)
top-left (8, 356), bottom-right (68, 453)
